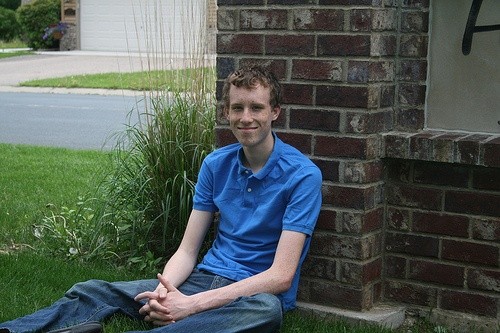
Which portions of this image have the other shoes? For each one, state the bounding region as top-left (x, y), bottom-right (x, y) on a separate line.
top-left (45, 321), bottom-right (105, 333)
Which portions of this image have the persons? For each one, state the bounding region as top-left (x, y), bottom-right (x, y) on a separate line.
top-left (0, 63), bottom-right (323, 333)
